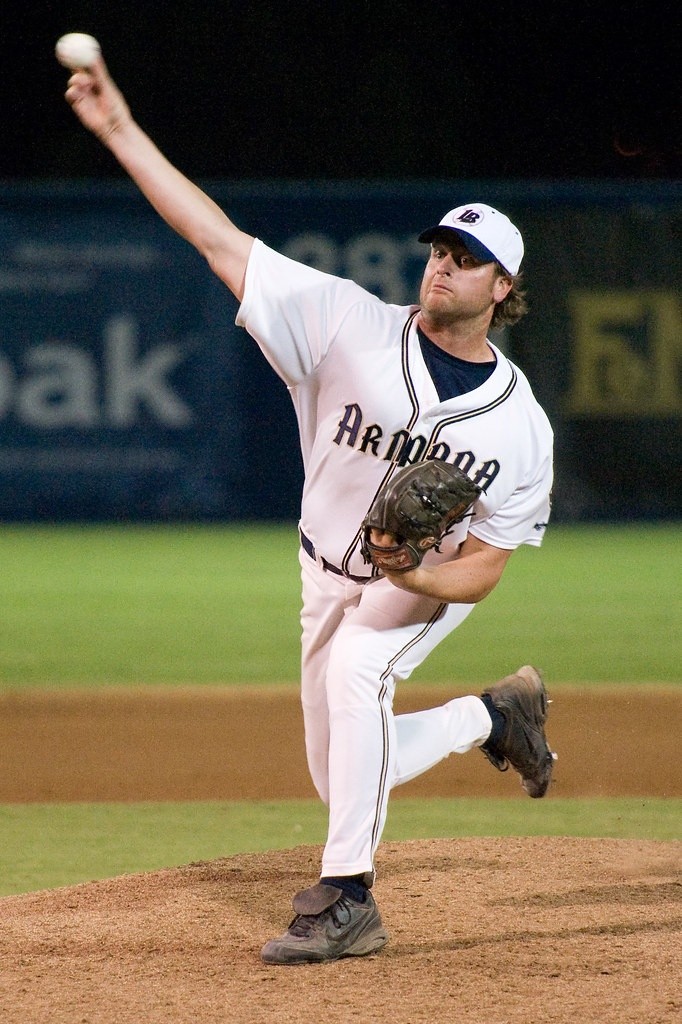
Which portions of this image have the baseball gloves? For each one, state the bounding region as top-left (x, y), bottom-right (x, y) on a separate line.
top-left (357, 456), bottom-right (488, 577)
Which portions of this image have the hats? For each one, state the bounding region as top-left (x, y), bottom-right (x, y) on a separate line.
top-left (419, 203), bottom-right (527, 277)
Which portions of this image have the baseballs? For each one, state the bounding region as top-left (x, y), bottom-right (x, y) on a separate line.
top-left (56, 32), bottom-right (100, 70)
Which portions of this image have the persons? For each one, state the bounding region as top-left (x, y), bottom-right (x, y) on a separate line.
top-left (65, 60), bottom-right (553, 966)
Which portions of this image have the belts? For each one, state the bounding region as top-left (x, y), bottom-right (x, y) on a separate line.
top-left (297, 529), bottom-right (371, 585)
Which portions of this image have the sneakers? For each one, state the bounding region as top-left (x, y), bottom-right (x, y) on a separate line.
top-left (260, 872), bottom-right (393, 967)
top-left (484, 660), bottom-right (554, 798)
